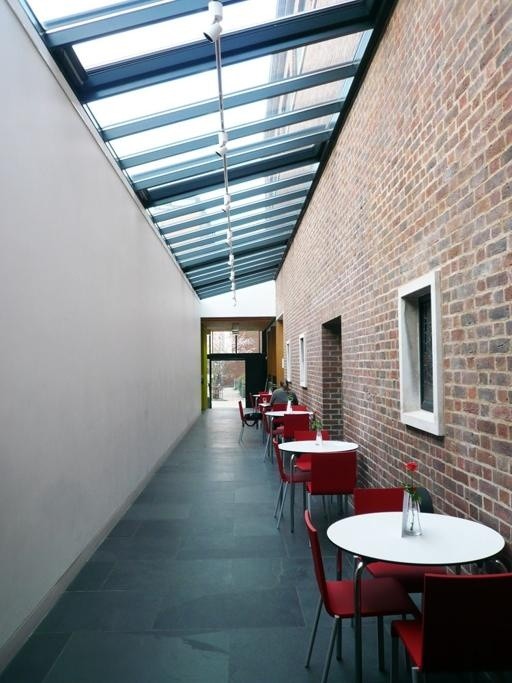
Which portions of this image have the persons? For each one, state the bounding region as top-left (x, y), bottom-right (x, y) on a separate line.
top-left (270, 381), bottom-right (288, 407)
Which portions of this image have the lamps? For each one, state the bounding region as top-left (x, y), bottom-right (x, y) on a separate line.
top-left (221, 195), bottom-right (231, 213)
top-left (225, 230), bottom-right (236, 291)
top-left (214, 129), bottom-right (230, 157)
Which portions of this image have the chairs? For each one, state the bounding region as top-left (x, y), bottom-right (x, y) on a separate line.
top-left (304, 510), bottom-right (422, 683)
top-left (390, 570), bottom-right (511, 683)
top-left (354, 486), bottom-right (446, 674)
top-left (239, 390), bottom-right (317, 438)
top-left (294, 429), bottom-right (331, 513)
top-left (262, 411), bottom-right (284, 462)
top-left (306, 450), bottom-right (357, 548)
top-left (272, 438), bottom-right (311, 529)
